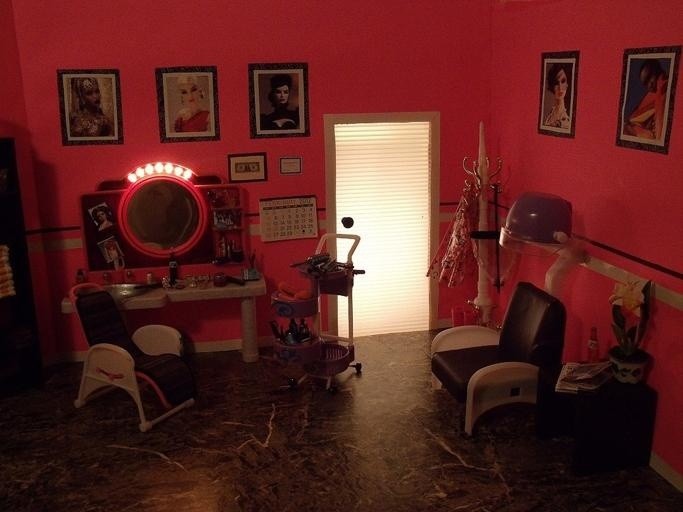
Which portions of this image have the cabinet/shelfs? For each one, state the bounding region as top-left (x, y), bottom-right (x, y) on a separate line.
top-left (0, 138), bottom-right (45, 400)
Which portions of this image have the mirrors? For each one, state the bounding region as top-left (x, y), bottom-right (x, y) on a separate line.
top-left (117, 162), bottom-right (210, 260)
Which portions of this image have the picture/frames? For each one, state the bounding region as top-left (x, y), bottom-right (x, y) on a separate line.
top-left (228, 152), bottom-right (267, 184)
top-left (280, 157), bottom-right (302, 174)
top-left (56, 69), bottom-right (124, 146)
top-left (616, 45), bottom-right (682, 155)
top-left (248, 63), bottom-right (311, 139)
top-left (536, 50), bottom-right (580, 140)
top-left (155, 66), bottom-right (220, 143)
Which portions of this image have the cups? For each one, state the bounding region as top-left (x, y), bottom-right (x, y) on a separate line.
top-left (197, 274), bottom-right (209, 290)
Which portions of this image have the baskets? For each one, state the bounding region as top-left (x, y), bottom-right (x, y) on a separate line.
top-left (301, 343), bottom-right (351, 376)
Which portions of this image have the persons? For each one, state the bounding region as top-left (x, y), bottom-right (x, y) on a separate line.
top-left (544, 62), bottom-right (570, 129)
top-left (103, 242), bottom-right (118, 261)
top-left (172, 76), bottom-right (211, 132)
top-left (68, 78), bottom-right (114, 136)
top-left (626, 64), bottom-right (669, 140)
top-left (91, 206), bottom-right (114, 231)
top-left (260, 75), bottom-right (300, 129)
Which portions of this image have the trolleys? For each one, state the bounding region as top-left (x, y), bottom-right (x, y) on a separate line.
top-left (271, 233), bottom-right (365, 393)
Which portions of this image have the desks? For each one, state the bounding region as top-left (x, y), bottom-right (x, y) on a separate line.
top-left (59, 273), bottom-right (268, 364)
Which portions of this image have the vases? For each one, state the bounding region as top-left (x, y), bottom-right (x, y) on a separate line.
top-left (608, 347), bottom-right (649, 383)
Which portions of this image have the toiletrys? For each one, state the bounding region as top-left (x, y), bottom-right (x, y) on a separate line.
top-left (76, 269), bottom-right (87, 284)
top-left (217, 232), bottom-right (235, 259)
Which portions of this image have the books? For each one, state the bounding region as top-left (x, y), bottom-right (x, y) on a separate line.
top-left (554, 359), bottom-right (613, 397)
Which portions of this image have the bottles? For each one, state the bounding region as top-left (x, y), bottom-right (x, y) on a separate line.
top-left (285, 317), bottom-right (310, 345)
top-left (586, 326), bottom-right (600, 367)
top-left (167, 252), bottom-right (178, 282)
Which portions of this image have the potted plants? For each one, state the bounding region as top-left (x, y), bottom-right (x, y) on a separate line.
top-left (605, 278), bottom-right (651, 355)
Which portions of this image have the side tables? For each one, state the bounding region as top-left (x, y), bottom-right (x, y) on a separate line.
top-left (536, 360), bottom-right (657, 479)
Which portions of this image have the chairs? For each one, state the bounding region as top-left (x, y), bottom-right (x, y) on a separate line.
top-left (427, 281), bottom-right (567, 436)
top-left (70, 282), bottom-right (199, 433)
